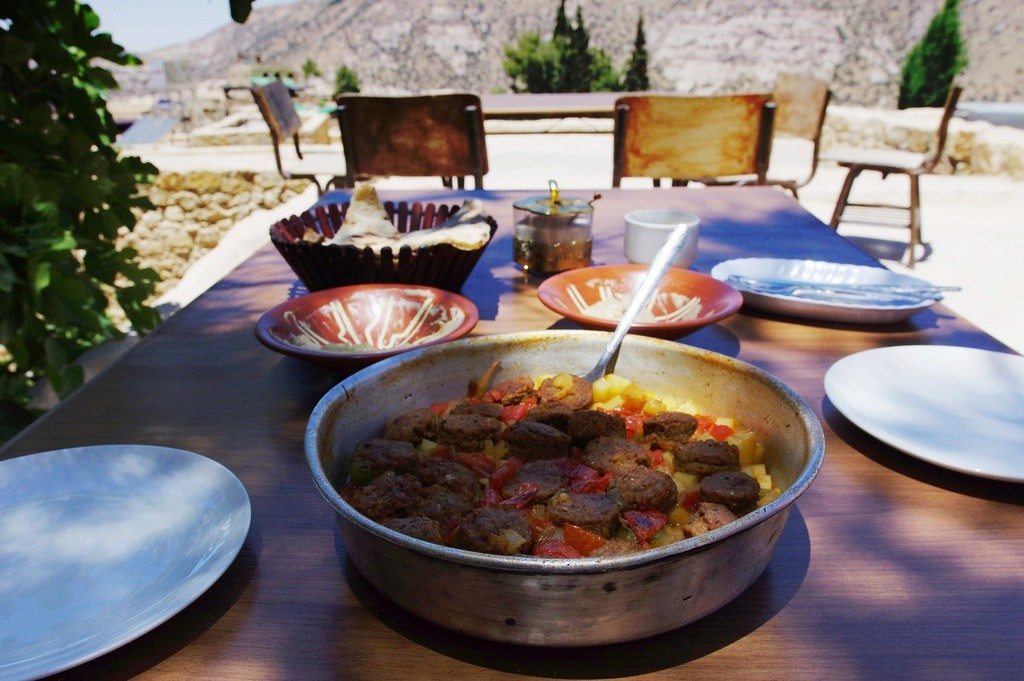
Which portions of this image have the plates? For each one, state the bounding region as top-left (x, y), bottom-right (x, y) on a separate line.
top-left (823, 344), bottom-right (1024, 484)
top-left (0, 442), bottom-right (252, 681)
top-left (710, 256), bottom-right (943, 320)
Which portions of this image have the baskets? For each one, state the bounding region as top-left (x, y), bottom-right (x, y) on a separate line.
top-left (269, 200), bottom-right (498, 292)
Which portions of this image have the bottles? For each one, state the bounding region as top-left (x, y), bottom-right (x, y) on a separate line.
top-left (513, 194), bottom-right (594, 278)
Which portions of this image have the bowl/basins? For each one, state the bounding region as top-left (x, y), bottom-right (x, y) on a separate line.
top-left (623, 207), bottom-right (701, 269)
top-left (254, 282), bottom-right (479, 359)
top-left (299, 328), bottom-right (827, 648)
top-left (537, 264), bottom-right (744, 341)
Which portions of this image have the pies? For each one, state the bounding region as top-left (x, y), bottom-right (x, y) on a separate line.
top-left (303, 183), bottom-right (492, 256)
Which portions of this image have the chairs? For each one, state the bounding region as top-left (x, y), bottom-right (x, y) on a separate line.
top-left (335, 91), bottom-right (490, 190)
top-left (822, 83), bottom-right (968, 269)
top-left (706, 73), bottom-right (831, 198)
top-left (250, 80), bottom-right (368, 197)
top-left (610, 94), bottom-right (772, 189)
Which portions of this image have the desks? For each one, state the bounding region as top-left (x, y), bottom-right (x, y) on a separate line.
top-left (0, 189), bottom-right (1024, 681)
top-left (443, 91), bottom-right (690, 188)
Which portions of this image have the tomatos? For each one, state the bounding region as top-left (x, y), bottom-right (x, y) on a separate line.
top-left (425, 393), bottom-right (735, 553)
